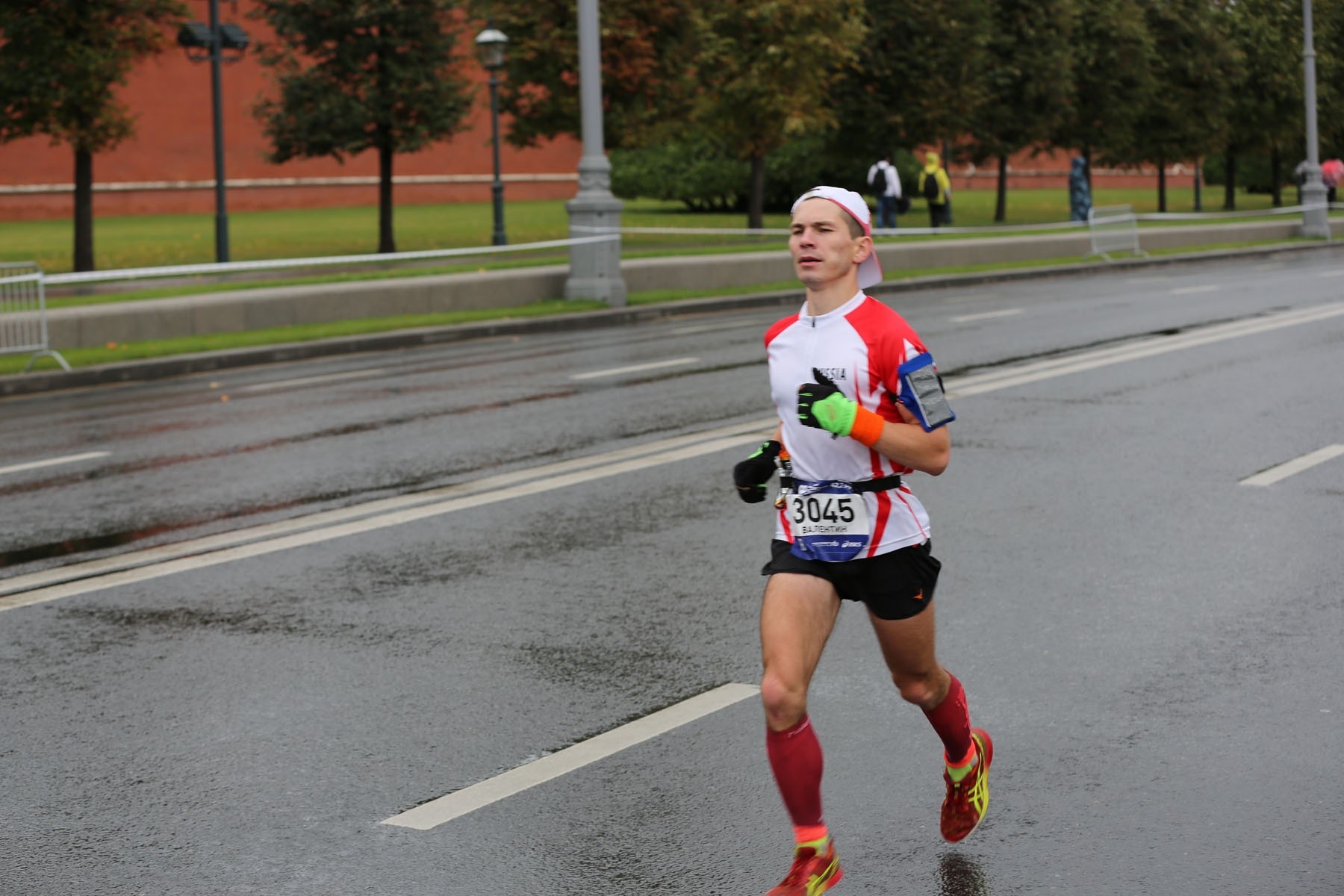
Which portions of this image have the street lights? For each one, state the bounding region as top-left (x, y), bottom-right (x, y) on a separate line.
top-left (471, 27), bottom-right (509, 245)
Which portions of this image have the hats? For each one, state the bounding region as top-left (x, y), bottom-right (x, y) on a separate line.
top-left (791, 186), bottom-right (884, 289)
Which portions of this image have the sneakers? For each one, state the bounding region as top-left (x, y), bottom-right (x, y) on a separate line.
top-left (940, 727), bottom-right (993, 844)
top-left (763, 838), bottom-right (844, 896)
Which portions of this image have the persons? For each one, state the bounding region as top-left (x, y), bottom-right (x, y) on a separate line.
top-left (917, 151), bottom-right (955, 228)
top-left (1293, 158), bottom-right (1344, 211)
top-left (866, 148), bottom-right (908, 230)
top-left (734, 187), bottom-right (993, 896)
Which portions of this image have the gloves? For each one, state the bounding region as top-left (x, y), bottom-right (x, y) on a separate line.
top-left (733, 440), bottom-right (782, 503)
top-left (796, 368), bottom-right (858, 439)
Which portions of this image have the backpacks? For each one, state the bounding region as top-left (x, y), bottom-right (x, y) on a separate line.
top-left (923, 167), bottom-right (940, 198)
top-left (873, 164), bottom-right (890, 193)
